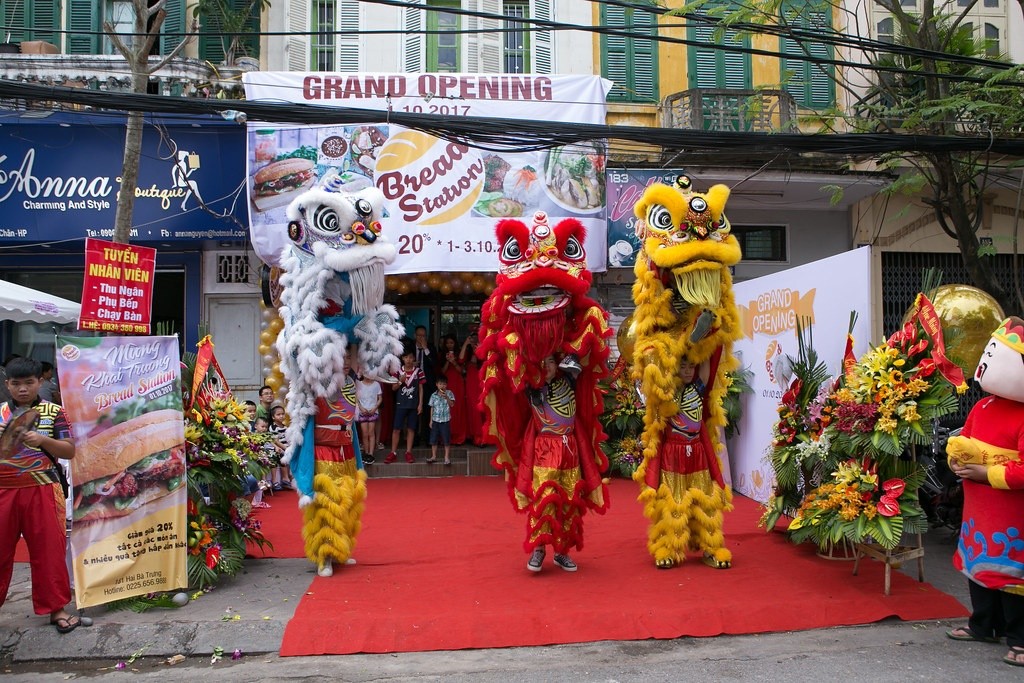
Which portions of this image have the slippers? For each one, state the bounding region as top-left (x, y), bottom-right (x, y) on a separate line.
top-left (52, 615), bottom-right (77, 633)
top-left (444, 460), bottom-right (451, 464)
top-left (945, 627), bottom-right (996, 642)
top-left (427, 458), bottom-right (436, 462)
top-left (1003, 646), bottom-right (1024, 666)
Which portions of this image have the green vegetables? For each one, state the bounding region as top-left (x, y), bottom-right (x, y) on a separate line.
top-left (551, 153), bottom-right (606, 193)
top-left (483, 159), bottom-right (502, 190)
top-left (274, 144), bottom-right (318, 164)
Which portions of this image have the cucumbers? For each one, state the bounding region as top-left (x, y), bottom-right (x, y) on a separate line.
top-left (475, 191), bottom-right (503, 214)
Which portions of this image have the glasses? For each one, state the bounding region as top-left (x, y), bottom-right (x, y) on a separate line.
top-left (261, 392), bottom-right (273, 396)
top-left (416, 334), bottom-right (426, 337)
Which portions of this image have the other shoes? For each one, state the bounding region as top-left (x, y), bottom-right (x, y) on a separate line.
top-left (282, 480), bottom-right (292, 487)
top-left (376, 442), bottom-right (384, 449)
top-left (689, 313), bottom-right (712, 344)
top-left (360, 444), bottom-right (363, 450)
top-left (271, 480), bottom-right (281, 489)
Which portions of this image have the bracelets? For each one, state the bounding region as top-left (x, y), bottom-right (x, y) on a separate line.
top-left (398, 382), bottom-right (402, 385)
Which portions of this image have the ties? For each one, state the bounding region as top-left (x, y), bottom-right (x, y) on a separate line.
top-left (417, 348), bottom-right (423, 367)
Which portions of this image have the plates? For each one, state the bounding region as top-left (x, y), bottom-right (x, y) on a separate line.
top-left (538, 150), bottom-right (606, 214)
top-left (472, 201), bottom-right (540, 217)
top-left (609, 245), bottom-right (622, 267)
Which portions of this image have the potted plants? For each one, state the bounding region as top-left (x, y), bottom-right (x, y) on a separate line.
top-left (184, 0), bottom-right (272, 79)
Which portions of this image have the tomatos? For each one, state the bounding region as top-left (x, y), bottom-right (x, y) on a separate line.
top-left (487, 199), bottom-right (524, 218)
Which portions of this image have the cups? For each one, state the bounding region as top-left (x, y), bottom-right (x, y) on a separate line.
top-left (615, 240), bottom-right (633, 262)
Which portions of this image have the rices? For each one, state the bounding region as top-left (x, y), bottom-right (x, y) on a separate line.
top-left (504, 163), bottom-right (544, 207)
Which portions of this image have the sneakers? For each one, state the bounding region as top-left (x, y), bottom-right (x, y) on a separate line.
top-left (318, 557), bottom-right (334, 577)
top-left (335, 558), bottom-right (356, 564)
top-left (384, 452), bottom-right (397, 462)
top-left (554, 554), bottom-right (577, 571)
top-left (558, 355), bottom-right (582, 373)
top-left (362, 452), bottom-right (375, 464)
top-left (405, 452), bottom-right (414, 463)
top-left (526, 550), bottom-right (545, 572)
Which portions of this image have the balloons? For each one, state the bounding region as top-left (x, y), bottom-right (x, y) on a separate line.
top-left (259, 299), bottom-right (291, 427)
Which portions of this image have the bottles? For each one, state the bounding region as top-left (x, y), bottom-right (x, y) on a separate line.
top-left (255, 129), bottom-right (277, 163)
top-left (99, 81), bottom-right (107, 91)
top-left (163, 82), bottom-right (171, 96)
top-left (218, 109), bottom-right (248, 123)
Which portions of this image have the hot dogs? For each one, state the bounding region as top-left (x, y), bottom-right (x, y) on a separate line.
top-left (71, 408), bottom-right (185, 522)
top-left (252, 157), bottom-right (316, 196)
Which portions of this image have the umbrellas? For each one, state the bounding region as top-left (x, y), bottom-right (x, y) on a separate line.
top-left (0, 279), bottom-right (81, 325)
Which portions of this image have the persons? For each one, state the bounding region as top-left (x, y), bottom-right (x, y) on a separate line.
top-left (358, 376), bottom-right (383, 464)
top-left (641, 356), bottom-right (734, 569)
top-left (384, 350), bottom-right (427, 464)
top-left (441, 332), bottom-right (484, 445)
top-left (409, 325), bottom-right (437, 382)
top-left (301, 342), bottom-right (369, 576)
top-left (523, 355), bottom-right (588, 572)
top-left (243, 386), bottom-right (296, 507)
top-left (945, 315), bottom-right (1024, 666)
top-left (427, 375), bottom-right (455, 465)
top-left (0, 353), bottom-right (79, 633)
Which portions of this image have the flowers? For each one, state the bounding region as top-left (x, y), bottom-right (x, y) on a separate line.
top-left (757, 259), bottom-right (955, 547)
top-left (599, 358), bottom-right (644, 478)
top-left (177, 334), bottom-right (278, 596)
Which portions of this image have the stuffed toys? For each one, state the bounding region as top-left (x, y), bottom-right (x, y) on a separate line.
top-left (281, 173), bottom-right (406, 462)
top-left (475, 211), bottom-right (614, 516)
top-left (628, 175), bottom-right (742, 454)
